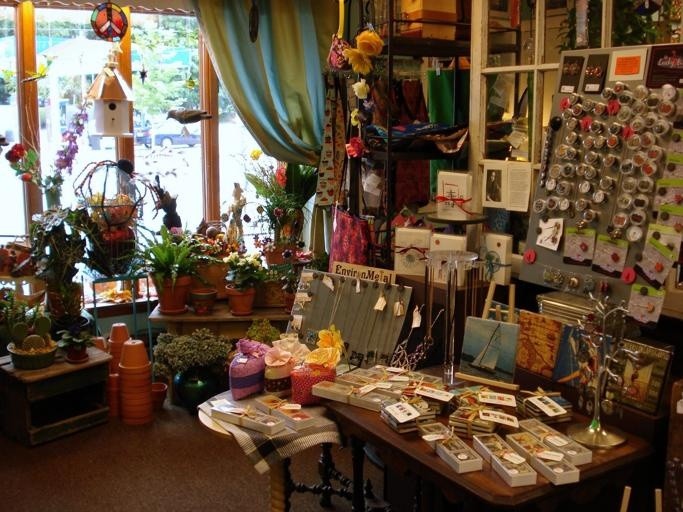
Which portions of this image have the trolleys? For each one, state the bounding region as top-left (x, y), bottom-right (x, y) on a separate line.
top-left (154, 121), bottom-right (202, 147)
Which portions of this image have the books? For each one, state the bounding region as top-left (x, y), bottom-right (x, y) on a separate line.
top-left (435, 170), bottom-right (474, 221)
top-left (393, 228), bottom-right (431, 276)
top-left (449, 407), bottom-right (505, 440)
top-left (480, 160), bottom-right (533, 213)
top-left (516, 393), bottom-right (574, 424)
top-left (536, 292), bottom-right (596, 328)
top-left (478, 232), bottom-right (514, 287)
top-left (428, 233), bottom-right (467, 287)
top-left (380, 402), bottom-right (443, 434)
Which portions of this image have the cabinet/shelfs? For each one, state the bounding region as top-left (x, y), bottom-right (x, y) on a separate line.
top-left (467, 0), bottom-right (681, 318)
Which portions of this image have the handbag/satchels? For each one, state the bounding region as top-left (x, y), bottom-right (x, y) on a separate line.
top-left (328, 203), bottom-right (368, 274)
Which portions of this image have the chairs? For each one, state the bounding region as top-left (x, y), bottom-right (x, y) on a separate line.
top-left (199, 268), bottom-right (412, 505)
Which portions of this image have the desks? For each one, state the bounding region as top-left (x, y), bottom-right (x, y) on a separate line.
top-left (314, 361), bottom-right (681, 512)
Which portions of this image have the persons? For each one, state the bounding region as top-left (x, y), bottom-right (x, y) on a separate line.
top-left (363, 163), bottom-right (385, 218)
top-left (61, 132), bottom-right (78, 176)
top-left (486, 170), bottom-right (502, 202)
